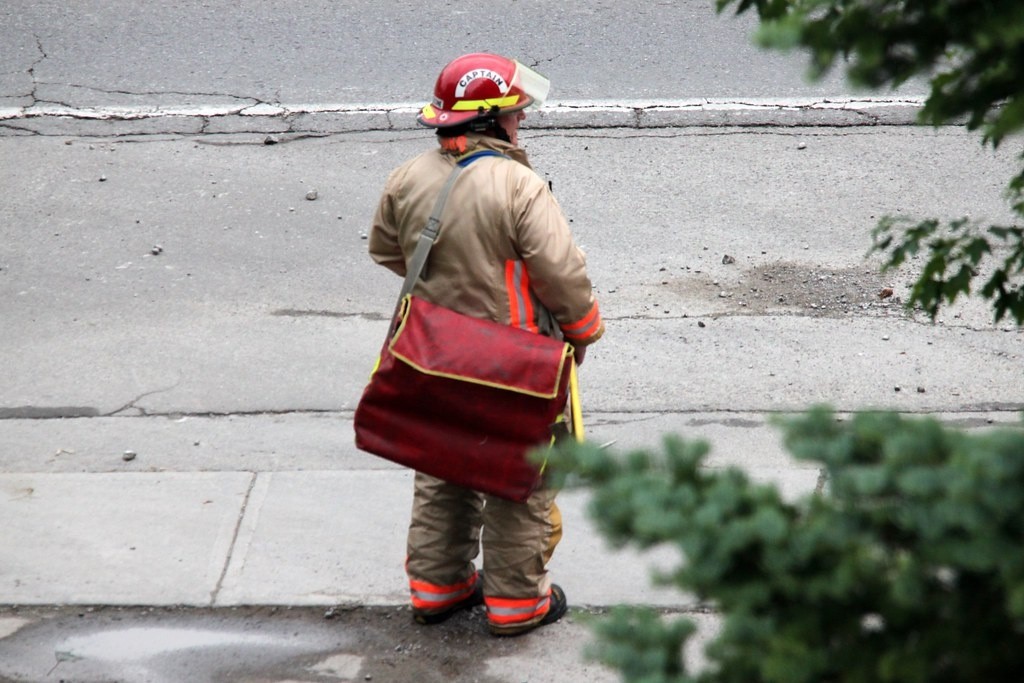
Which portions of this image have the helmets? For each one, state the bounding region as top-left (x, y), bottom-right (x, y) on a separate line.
top-left (416, 53), bottom-right (549, 127)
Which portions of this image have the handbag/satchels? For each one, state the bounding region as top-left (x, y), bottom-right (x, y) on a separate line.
top-left (352, 296), bottom-right (581, 503)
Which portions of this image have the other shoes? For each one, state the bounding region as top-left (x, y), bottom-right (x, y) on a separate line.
top-left (489, 583), bottom-right (567, 636)
top-left (414, 569), bottom-right (485, 624)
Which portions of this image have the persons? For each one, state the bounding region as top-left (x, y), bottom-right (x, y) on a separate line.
top-left (369, 54), bottom-right (605, 634)
